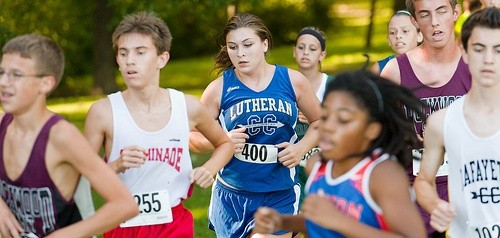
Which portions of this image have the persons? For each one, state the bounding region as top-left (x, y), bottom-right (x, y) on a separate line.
top-left (413, 5), bottom-right (500, 238)
top-left (286, 25), bottom-right (335, 238)
top-left (379, 0), bottom-right (473, 238)
top-left (455, 0), bottom-right (484, 47)
top-left (187, 12), bottom-right (323, 238)
top-left (253, 70), bottom-right (429, 238)
top-left (0, 33), bottom-right (139, 238)
top-left (83, 10), bottom-right (235, 238)
top-left (368, 9), bottom-right (424, 79)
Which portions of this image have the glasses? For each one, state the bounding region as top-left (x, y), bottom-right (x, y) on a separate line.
top-left (0, 67), bottom-right (52, 84)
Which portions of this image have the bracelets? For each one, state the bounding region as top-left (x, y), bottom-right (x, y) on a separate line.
top-left (117, 160), bottom-right (125, 174)
